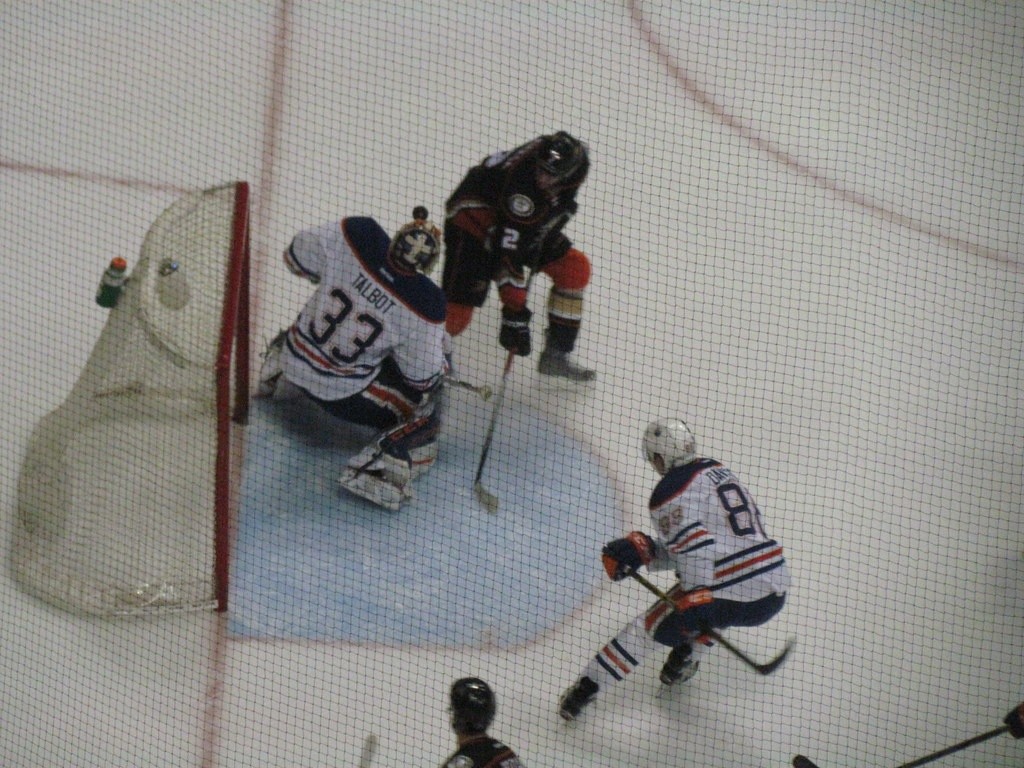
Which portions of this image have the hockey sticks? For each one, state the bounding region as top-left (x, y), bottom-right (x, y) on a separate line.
top-left (474, 243), bottom-right (547, 512)
top-left (792, 725), bottom-right (1007, 768)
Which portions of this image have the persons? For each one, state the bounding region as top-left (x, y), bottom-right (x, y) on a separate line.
top-left (440, 678), bottom-right (524, 768)
top-left (439, 131), bottom-right (600, 381)
top-left (556, 415), bottom-right (791, 721)
top-left (252, 213), bottom-right (450, 509)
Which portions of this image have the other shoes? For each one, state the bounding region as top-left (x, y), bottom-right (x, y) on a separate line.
top-left (537, 348), bottom-right (596, 381)
top-left (251, 379), bottom-right (273, 400)
top-left (336, 468), bottom-right (402, 510)
top-left (558, 676), bottom-right (599, 722)
top-left (656, 652), bottom-right (697, 697)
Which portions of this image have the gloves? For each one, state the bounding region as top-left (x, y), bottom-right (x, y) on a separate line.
top-left (601, 531), bottom-right (656, 581)
top-left (499, 306), bottom-right (532, 356)
top-left (677, 587), bottom-right (714, 647)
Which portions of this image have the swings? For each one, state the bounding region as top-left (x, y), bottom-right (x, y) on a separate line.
top-left (624, 565), bottom-right (796, 675)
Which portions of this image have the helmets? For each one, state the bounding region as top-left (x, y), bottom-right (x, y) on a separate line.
top-left (388, 218), bottom-right (440, 276)
top-left (535, 131), bottom-right (580, 177)
top-left (642, 418), bottom-right (696, 468)
top-left (451, 677), bottom-right (495, 736)
